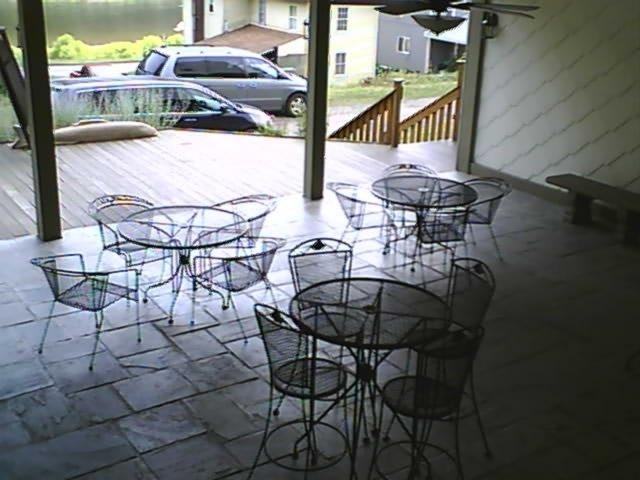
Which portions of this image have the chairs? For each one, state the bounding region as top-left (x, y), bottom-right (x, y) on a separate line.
top-left (383, 164), bottom-right (439, 254)
top-left (368, 325), bottom-right (486, 479)
top-left (182, 193), bottom-right (276, 296)
top-left (88, 196), bottom-right (179, 308)
top-left (30, 254), bottom-right (142, 372)
top-left (326, 180), bottom-right (398, 256)
top-left (272, 237), bottom-right (371, 443)
top-left (191, 234), bottom-right (285, 345)
top-left (248, 304), bottom-right (351, 479)
top-left (411, 204), bottom-right (471, 292)
top-left (383, 256), bottom-right (496, 460)
top-left (431, 177), bottom-right (511, 264)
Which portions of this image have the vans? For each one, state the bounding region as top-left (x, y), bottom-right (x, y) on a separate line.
top-left (133, 45), bottom-right (308, 118)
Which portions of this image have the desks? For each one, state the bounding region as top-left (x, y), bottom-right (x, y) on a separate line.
top-left (293, 277), bottom-right (451, 476)
top-left (119, 206), bottom-right (249, 325)
top-left (371, 175), bottom-right (478, 270)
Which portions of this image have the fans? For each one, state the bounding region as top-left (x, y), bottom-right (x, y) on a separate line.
top-left (329, 1), bottom-right (540, 37)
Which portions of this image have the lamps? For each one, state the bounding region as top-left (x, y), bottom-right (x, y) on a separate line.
top-left (483, 12), bottom-right (498, 38)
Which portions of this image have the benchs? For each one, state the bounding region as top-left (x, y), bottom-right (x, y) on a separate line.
top-left (546, 172), bottom-right (639, 244)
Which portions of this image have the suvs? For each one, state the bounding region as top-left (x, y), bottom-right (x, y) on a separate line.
top-left (56, 78), bottom-right (277, 135)
